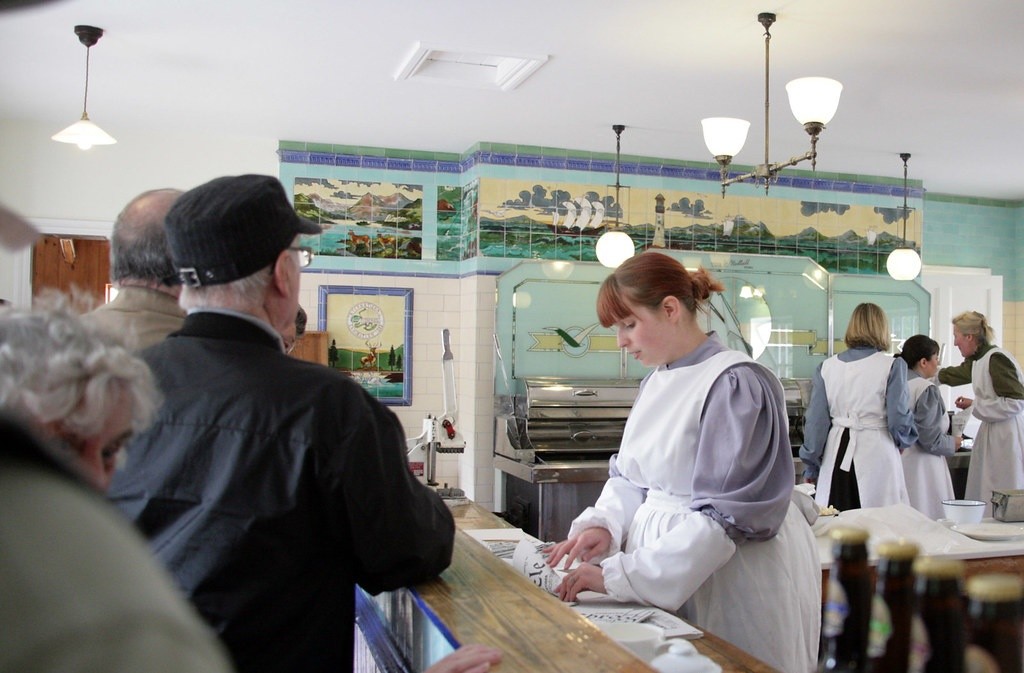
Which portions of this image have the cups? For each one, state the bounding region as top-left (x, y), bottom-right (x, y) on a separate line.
top-left (596, 622), bottom-right (697, 664)
top-left (651, 643), bottom-right (723, 673)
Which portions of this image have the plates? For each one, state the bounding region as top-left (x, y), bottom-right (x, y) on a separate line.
top-left (950, 524), bottom-right (1024, 541)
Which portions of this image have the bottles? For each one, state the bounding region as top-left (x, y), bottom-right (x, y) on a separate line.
top-left (811, 524), bottom-right (1023, 673)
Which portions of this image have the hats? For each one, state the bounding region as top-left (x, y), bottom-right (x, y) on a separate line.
top-left (164, 173), bottom-right (323, 288)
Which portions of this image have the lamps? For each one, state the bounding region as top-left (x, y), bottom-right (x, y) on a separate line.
top-left (51, 24), bottom-right (117, 150)
top-left (697, 13), bottom-right (841, 198)
top-left (886, 153), bottom-right (922, 282)
top-left (595, 125), bottom-right (636, 270)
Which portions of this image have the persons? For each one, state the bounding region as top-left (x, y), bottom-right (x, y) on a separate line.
top-left (542, 252), bottom-right (820, 673)
top-left (109, 174), bottom-right (455, 672)
top-left (81, 189), bottom-right (189, 354)
top-left (799, 302), bottom-right (920, 512)
top-left (894, 334), bottom-right (963, 520)
top-left (0, 405), bottom-right (232, 672)
top-left (927, 311), bottom-right (1024, 517)
top-left (0, 285), bottom-right (503, 673)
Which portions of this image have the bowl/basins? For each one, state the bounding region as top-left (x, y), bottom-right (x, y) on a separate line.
top-left (942, 500), bottom-right (987, 525)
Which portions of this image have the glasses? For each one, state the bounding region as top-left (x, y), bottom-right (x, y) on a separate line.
top-left (286, 245), bottom-right (313, 268)
